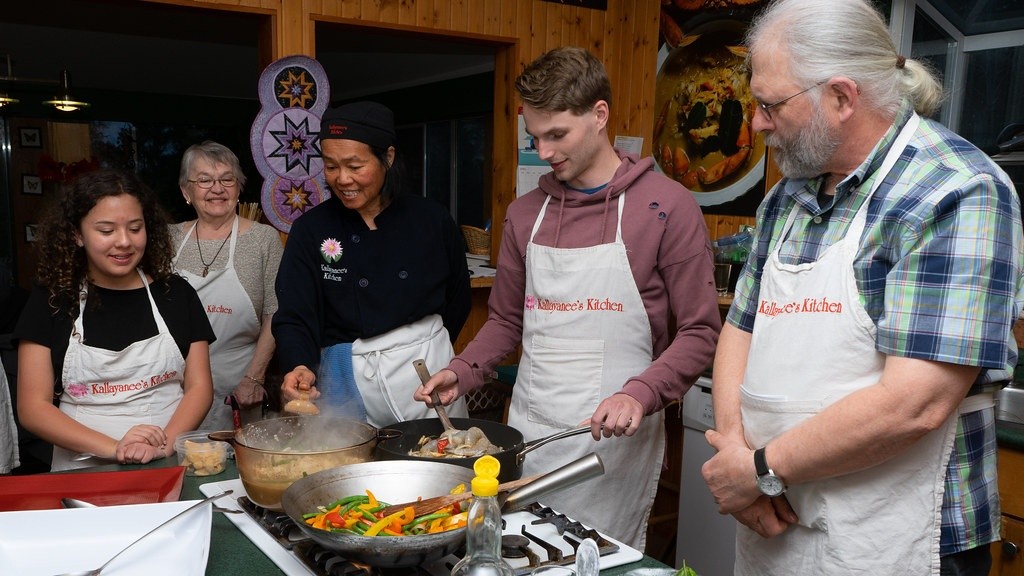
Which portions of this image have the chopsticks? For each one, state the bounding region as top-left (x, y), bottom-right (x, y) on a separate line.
top-left (238, 202), bottom-right (262, 222)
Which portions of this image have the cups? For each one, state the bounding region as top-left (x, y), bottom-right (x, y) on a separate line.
top-left (714, 264), bottom-right (732, 297)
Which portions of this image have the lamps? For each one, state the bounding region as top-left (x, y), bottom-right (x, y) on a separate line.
top-left (0, 89), bottom-right (19, 107)
top-left (42, 66), bottom-right (91, 112)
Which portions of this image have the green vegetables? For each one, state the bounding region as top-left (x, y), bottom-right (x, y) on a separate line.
top-left (683, 99), bottom-right (743, 157)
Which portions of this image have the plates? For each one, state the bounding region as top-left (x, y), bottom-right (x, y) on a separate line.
top-left (618, 567), bottom-right (677, 576)
top-left (0, 500), bottom-right (213, 576)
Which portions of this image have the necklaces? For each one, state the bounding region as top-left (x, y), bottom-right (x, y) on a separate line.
top-left (196, 221), bottom-right (231, 277)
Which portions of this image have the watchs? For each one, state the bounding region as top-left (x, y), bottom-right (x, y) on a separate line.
top-left (754, 448), bottom-right (788, 497)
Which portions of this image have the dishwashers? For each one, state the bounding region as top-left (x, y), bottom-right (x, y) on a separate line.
top-left (675, 377), bottom-right (737, 575)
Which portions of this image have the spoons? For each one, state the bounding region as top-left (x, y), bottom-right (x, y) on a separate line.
top-left (62, 497), bottom-right (244, 514)
top-left (69, 452), bottom-right (114, 461)
top-left (54, 490), bottom-right (234, 576)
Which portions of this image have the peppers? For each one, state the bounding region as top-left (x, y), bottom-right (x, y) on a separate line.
top-left (313, 483), bottom-right (484, 537)
top-left (306, 518), bottom-right (316, 524)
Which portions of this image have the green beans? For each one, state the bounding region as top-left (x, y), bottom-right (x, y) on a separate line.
top-left (303, 495), bottom-right (450, 537)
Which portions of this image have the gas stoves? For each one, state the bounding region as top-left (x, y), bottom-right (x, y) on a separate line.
top-left (198, 478), bottom-right (643, 576)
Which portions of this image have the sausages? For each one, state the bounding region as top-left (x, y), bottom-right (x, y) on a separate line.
top-left (421, 427), bottom-right (505, 460)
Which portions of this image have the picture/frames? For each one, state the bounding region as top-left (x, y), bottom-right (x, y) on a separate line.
top-left (18, 127), bottom-right (43, 148)
top-left (21, 173), bottom-right (44, 196)
top-left (23, 222), bottom-right (38, 243)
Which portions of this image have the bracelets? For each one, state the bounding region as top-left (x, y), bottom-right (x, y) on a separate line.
top-left (245, 376), bottom-right (263, 384)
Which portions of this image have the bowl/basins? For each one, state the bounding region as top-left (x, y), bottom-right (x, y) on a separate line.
top-left (173, 430), bottom-right (229, 476)
top-left (208, 415), bottom-right (405, 513)
top-left (652, 11), bottom-right (766, 205)
top-left (715, 258), bottom-right (745, 293)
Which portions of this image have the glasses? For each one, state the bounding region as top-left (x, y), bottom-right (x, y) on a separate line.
top-left (185, 176), bottom-right (238, 189)
top-left (757, 76), bottom-right (857, 121)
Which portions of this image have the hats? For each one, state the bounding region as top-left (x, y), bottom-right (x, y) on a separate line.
top-left (319, 102), bottom-right (398, 153)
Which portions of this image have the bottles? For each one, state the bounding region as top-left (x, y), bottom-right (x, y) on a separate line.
top-left (449, 455), bottom-right (517, 576)
top-left (531, 538), bottom-right (600, 576)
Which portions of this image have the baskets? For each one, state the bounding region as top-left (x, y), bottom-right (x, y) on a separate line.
top-left (460, 225), bottom-right (491, 255)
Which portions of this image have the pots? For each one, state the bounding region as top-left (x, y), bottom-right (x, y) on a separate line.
top-left (372, 417), bottom-right (606, 484)
top-left (282, 452), bottom-right (606, 568)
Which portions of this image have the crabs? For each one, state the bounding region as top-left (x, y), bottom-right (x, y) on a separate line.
top-left (485, 444), bottom-right (500, 454)
top-left (654, 0), bottom-right (773, 189)
top-left (447, 449), bottom-right (485, 456)
top-left (452, 430), bottom-right (470, 445)
top-left (420, 440), bottom-right (438, 453)
top-left (464, 427), bottom-right (488, 448)
top-left (472, 438), bottom-right (491, 450)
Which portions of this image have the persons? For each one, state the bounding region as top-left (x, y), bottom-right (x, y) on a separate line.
top-left (15, 171), bottom-right (217, 469)
top-left (701, 0), bottom-right (1024, 576)
top-left (271, 100), bottom-right (472, 415)
top-left (414, 47), bottom-right (721, 556)
top-left (168, 141), bottom-right (283, 433)
